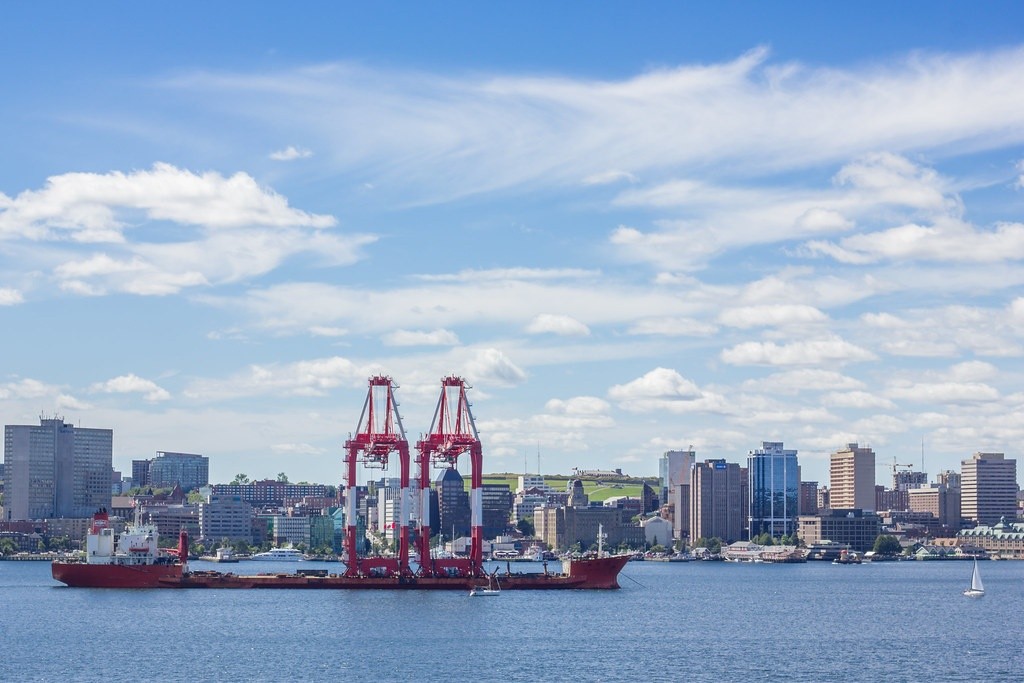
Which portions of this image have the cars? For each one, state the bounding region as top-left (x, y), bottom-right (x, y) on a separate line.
top-left (32, 551), bottom-right (40, 554)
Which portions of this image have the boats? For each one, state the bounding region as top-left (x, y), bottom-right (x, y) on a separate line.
top-left (324, 555), bottom-right (336, 562)
top-left (763, 547), bottom-right (807, 564)
top-left (468, 586), bottom-right (501, 596)
top-left (51, 374), bottom-right (641, 590)
top-left (305, 557), bottom-right (325, 562)
top-left (249, 548), bottom-right (304, 562)
top-left (668, 554), bottom-right (691, 562)
top-left (492, 543), bottom-right (543, 563)
top-left (832, 550), bottom-right (863, 564)
top-left (702, 556), bottom-right (724, 562)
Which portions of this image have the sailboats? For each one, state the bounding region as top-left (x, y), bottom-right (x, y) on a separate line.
top-left (963, 555), bottom-right (984, 598)
